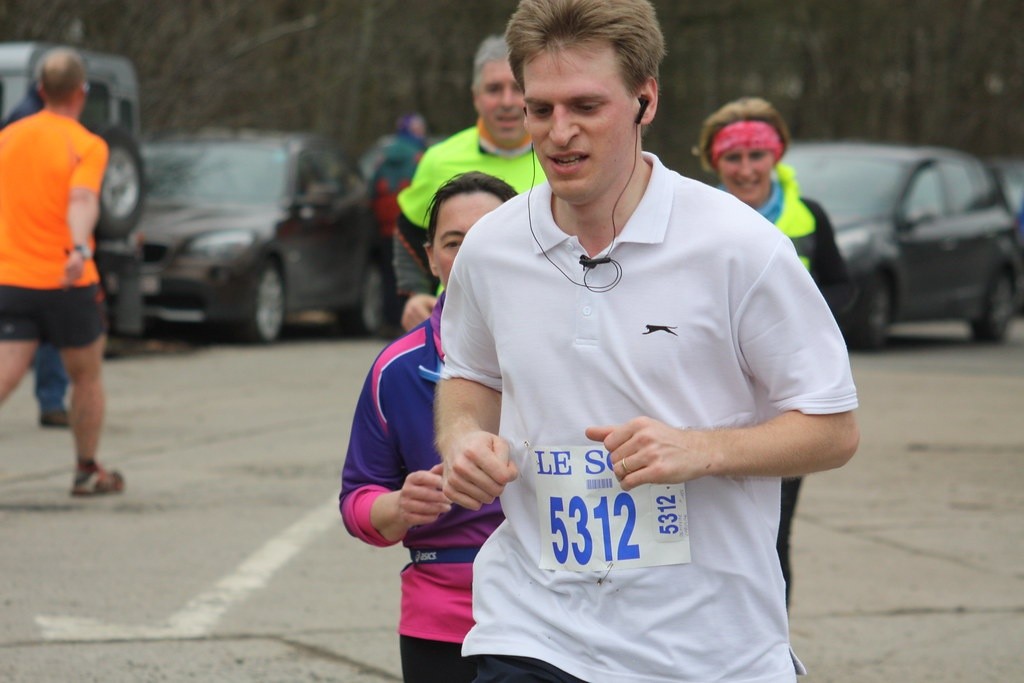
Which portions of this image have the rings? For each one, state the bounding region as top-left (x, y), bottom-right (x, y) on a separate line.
top-left (622, 458), bottom-right (632, 474)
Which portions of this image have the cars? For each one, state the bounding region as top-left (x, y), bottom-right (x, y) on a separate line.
top-left (120, 125), bottom-right (404, 347)
top-left (776, 140), bottom-right (1024, 356)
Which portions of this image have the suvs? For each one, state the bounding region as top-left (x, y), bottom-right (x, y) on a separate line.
top-left (1, 41), bottom-right (150, 360)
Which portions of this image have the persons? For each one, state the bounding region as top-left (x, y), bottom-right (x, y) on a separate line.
top-left (0, 53), bottom-right (72, 428)
top-left (700, 99), bottom-right (847, 612)
top-left (339, 171), bottom-right (518, 683)
top-left (437, 0), bottom-right (860, 683)
top-left (362, 114), bottom-right (439, 328)
top-left (0, 49), bottom-right (122, 497)
top-left (393, 35), bottom-right (545, 330)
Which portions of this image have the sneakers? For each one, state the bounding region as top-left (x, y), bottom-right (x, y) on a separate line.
top-left (68, 463), bottom-right (124, 498)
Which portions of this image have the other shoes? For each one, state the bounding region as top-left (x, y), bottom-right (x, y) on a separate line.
top-left (39, 408), bottom-right (69, 428)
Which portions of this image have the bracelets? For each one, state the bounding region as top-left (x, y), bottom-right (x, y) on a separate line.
top-left (74, 244), bottom-right (90, 259)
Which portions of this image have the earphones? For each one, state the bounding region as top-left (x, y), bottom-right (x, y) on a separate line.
top-left (635, 97), bottom-right (650, 124)
